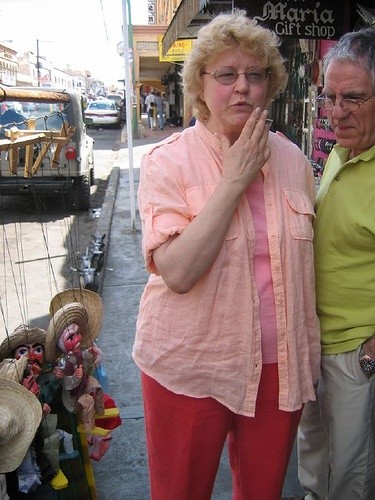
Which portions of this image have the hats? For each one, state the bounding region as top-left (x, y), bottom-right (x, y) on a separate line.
top-left (0, 379), bottom-right (42, 473)
top-left (50, 288), bottom-right (102, 350)
top-left (0, 324), bottom-right (47, 356)
top-left (0, 354), bottom-right (27, 382)
top-left (45, 302), bottom-right (90, 363)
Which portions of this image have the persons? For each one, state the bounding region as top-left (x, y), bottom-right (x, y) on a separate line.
top-left (81, 92), bottom-right (107, 109)
top-left (131, 11), bottom-right (322, 500)
top-left (140, 91), bottom-right (164, 130)
top-left (297, 28), bottom-right (375, 500)
top-left (0, 303), bottom-right (104, 490)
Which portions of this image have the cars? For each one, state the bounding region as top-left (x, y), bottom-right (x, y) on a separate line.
top-left (83, 100), bottom-right (122, 128)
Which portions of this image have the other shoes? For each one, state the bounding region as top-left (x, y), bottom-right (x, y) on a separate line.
top-left (160, 128), bottom-right (163, 130)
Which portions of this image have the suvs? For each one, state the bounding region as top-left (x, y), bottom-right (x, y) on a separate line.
top-left (0, 86), bottom-right (95, 210)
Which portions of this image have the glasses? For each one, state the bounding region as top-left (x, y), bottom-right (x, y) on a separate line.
top-left (202, 66), bottom-right (272, 85)
top-left (316, 92), bottom-right (374, 112)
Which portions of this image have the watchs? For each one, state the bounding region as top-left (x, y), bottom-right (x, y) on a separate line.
top-left (359, 349), bottom-right (375, 374)
